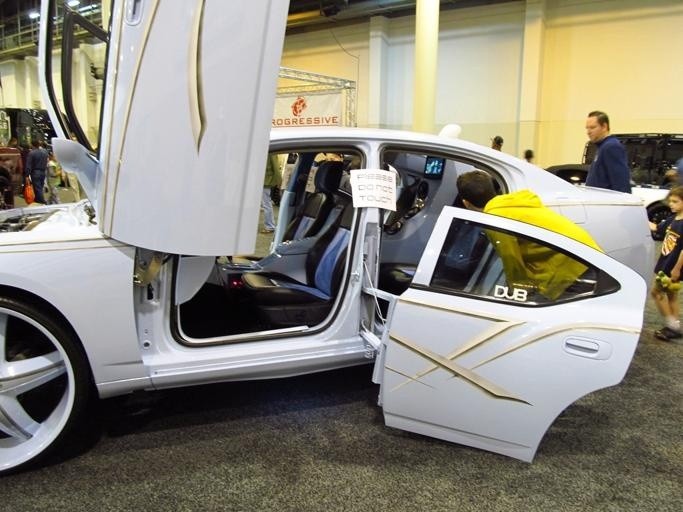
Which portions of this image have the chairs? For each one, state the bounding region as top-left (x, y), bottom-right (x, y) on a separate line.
top-left (212, 159), bottom-right (343, 288)
top-left (242, 202), bottom-right (354, 326)
top-left (383, 163), bottom-right (420, 228)
top-left (378, 177), bottom-right (501, 323)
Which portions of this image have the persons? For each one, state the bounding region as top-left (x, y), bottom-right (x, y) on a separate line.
top-left (489, 135), bottom-right (503, 152)
top-left (648, 184), bottom-right (683, 340)
top-left (663, 157), bottom-right (683, 187)
top-left (455, 170), bottom-right (604, 303)
top-left (523, 149), bottom-right (534, 162)
top-left (322, 151), bottom-right (351, 202)
top-left (261, 154), bottom-right (283, 234)
top-left (6, 132), bottom-right (81, 205)
top-left (585, 111), bottom-right (631, 193)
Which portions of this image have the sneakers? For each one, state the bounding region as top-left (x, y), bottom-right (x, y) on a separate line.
top-left (654, 326), bottom-right (683, 340)
